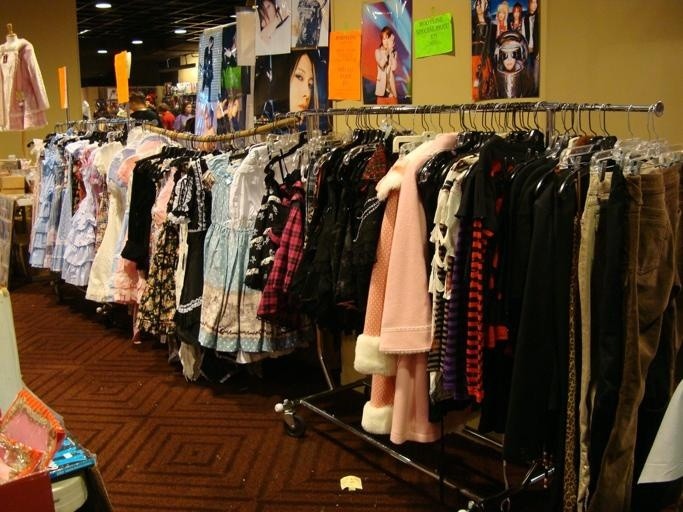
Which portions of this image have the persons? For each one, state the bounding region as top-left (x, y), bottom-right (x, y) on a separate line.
top-left (199, 34), bottom-right (217, 104)
top-left (373, 26), bottom-right (399, 105)
top-left (509, 3), bottom-right (523, 33)
top-left (1, 22), bottom-right (53, 131)
top-left (202, 104), bottom-right (215, 135)
top-left (256, 0), bottom-right (292, 44)
top-left (223, 36), bottom-right (250, 92)
top-left (260, 51), bottom-right (320, 131)
top-left (83, 88), bottom-right (196, 133)
top-left (296, 0), bottom-right (324, 48)
top-left (472, 1), bottom-right (494, 101)
top-left (216, 94), bottom-right (243, 134)
top-left (493, 1), bottom-right (512, 37)
top-left (523, 0), bottom-right (541, 81)
top-left (490, 30), bottom-right (529, 98)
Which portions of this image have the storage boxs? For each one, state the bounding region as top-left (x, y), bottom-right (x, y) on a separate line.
top-left (0, 176), bottom-right (26, 194)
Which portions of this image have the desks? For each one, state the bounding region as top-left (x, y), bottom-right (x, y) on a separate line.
top-left (1, 192), bottom-right (34, 280)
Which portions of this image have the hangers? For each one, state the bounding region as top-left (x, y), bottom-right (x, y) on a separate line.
top-left (27, 117), bottom-right (138, 147)
top-left (291, 102), bottom-right (683, 181)
top-left (141, 111), bottom-right (300, 158)
